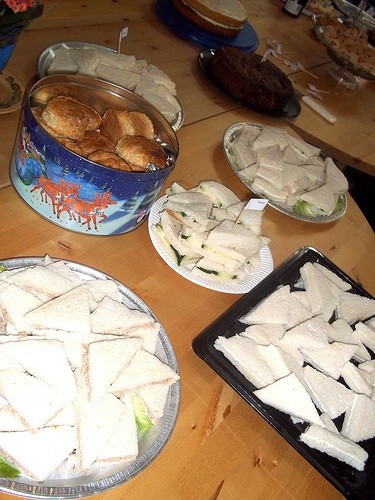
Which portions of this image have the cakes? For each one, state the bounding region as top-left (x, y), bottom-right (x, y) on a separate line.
top-left (173, 0), bottom-right (247, 39)
top-left (209, 45), bottom-right (295, 113)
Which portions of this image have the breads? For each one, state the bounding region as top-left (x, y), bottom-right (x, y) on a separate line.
top-left (305, 0), bottom-right (375, 76)
top-left (32, 95), bottom-right (170, 172)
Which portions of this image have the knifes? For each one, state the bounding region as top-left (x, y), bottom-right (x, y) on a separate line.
top-left (294, 87), bottom-right (337, 123)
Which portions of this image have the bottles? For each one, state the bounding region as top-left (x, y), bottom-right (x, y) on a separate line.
top-left (282, 0), bottom-right (308, 18)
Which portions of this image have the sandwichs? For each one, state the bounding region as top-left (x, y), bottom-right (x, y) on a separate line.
top-left (0, 118), bottom-right (375, 481)
top-left (49, 48), bottom-right (181, 123)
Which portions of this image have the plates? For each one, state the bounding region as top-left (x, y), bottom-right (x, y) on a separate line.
top-left (224, 122), bottom-right (347, 224)
top-left (0, 256), bottom-right (179, 499)
top-left (148, 188), bottom-right (275, 294)
top-left (196, 47), bottom-right (301, 120)
top-left (38, 41), bottom-right (185, 131)
top-left (0, 67), bottom-right (26, 114)
top-left (191, 246), bottom-right (375, 500)
top-left (146, 1), bottom-right (259, 54)
